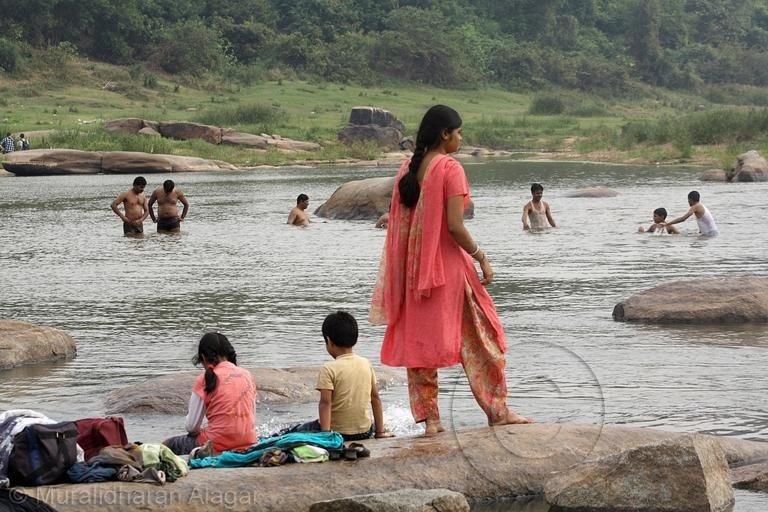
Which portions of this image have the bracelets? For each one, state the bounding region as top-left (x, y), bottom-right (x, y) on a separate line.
top-left (468, 243), bottom-right (480, 257)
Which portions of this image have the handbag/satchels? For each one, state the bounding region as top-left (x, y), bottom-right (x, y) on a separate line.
top-left (75, 415), bottom-right (128, 463)
top-left (7, 419), bottom-right (80, 487)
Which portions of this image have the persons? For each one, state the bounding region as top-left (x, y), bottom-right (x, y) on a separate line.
top-left (110, 176), bottom-right (149, 236)
top-left (286, 193), bottom-right (328, 226)
top-left (522, 184), bottom-right (556, 229)
top-left (279, 310), bottom-right (397, 439)
top-left (376, 202), bottom-right (391, 229)
top-left (368, 104), bottom-right (537, 435)
top-left (0, 133), bottom-right (15, 153)
top-left (16, 133), bottom-right (30, 150)
top-left (638, 208), bottom-right (679, 235)
top-left (149, 178), bottom-right (189, 233)
top-left (159, 331), bottom-right (258, 455)
top-left (659, 191), bottom-right (720, 236)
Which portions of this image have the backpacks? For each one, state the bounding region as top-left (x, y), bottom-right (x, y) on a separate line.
top-left (21, 139), bottom-right (29, 150)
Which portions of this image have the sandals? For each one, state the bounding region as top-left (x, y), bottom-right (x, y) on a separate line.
top-left (327, 444), bottom-right (346, 460)
top-left (342, 443), bottom-right (371, 460)
